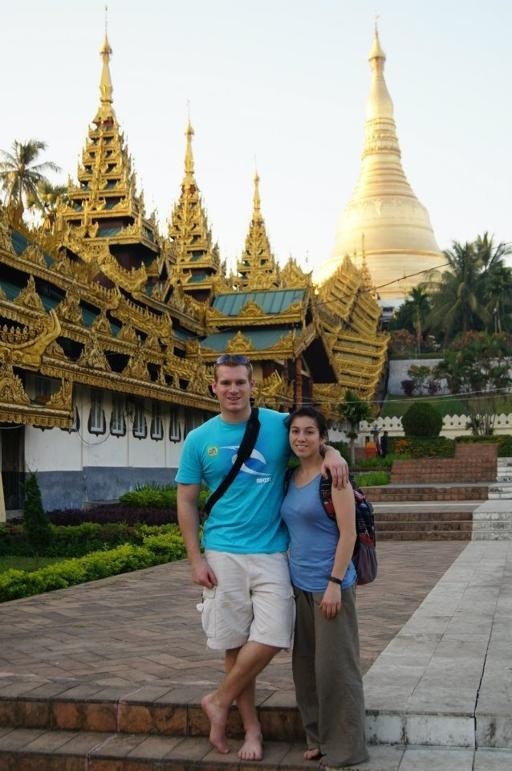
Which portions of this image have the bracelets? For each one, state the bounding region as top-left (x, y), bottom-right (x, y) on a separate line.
top-left (328, 575), bottom-right (343, 586)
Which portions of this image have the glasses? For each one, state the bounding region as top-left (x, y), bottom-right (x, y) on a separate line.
top-left (215, 353), bottom-right (251, 365)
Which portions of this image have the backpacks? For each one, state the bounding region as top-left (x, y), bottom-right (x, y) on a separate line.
top-left (283, 465), bottom-right (378, 586)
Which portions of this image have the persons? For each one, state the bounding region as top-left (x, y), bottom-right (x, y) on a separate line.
top-left (173, 354), bottom-right (349, 762)
top-left (276, 406), bottom-right (370, 769)
top-left (363, 430), bottom-right (388, 459)
